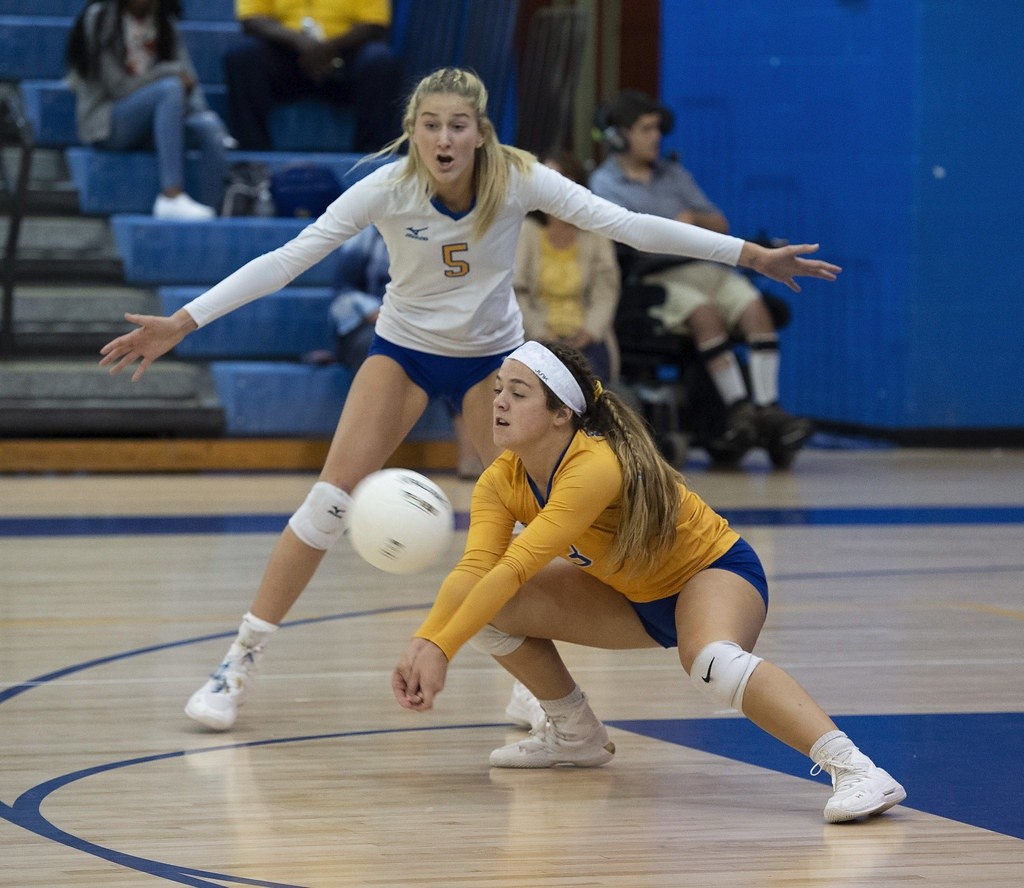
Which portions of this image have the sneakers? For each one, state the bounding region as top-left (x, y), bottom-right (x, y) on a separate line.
top-left (184, 639), bottom-right (264, 731)
top-left (504, 679), bottom-right (545, 729)
top-left (810, 748), bottom-right (907, 825)
top-left (489, 691), bottom-right (615, 770)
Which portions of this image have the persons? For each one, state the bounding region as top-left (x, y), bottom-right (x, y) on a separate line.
top-left (589, 97), bottom-right (811, 446)
top-left (513, 148), bottom-right (621, 386)
top-left (333, 224), bottom-right (487, 479)
top-left (222, 0), bottom-right (403, 154)
top-left (68, 0), bottom-right (237, 221)
top-left (391, 342), bottom-right (908, 822)
top-left (98, 68), bottom-right (842, 734)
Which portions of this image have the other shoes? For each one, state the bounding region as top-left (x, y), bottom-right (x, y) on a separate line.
top-left (761, 405), bottom-right (811, 442)
top-left (153, 192), bottom-right (217, 221)
top-left (724, 401), bottom-right (759, 440)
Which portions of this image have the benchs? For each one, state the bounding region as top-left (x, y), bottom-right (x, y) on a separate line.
top-left (0, 0), bottom-right (458, 440)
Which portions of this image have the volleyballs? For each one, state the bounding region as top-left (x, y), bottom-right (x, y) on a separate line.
top-left (346, 467), bottom-right (455, 575)
